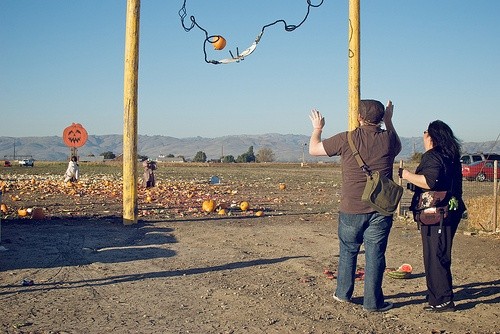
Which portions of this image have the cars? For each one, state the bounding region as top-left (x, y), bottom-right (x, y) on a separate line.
top-left (462, 159), bottom-right (500, 182)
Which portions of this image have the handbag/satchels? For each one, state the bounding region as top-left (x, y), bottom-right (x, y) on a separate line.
top-left (414, 191), bottom-right (450, 234)
top-left (362, 169), bottom-right (403, 216)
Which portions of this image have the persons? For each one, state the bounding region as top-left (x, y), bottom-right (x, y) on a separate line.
top-left (65, 156), bottom-right (78, 182)
top-left (309, 100), bottom-right (402, 310)
top-left (398, 120), bottom-right (466, 311)
top-left (143, 157), bottom-right (155, 187)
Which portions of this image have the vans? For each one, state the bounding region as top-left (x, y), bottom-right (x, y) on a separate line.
top-left (461, 152), bottom-right (500, 167)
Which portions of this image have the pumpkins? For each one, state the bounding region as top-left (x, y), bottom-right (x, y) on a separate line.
top-left (202, 199), bottom-right (265, 216)
top-left (0, 178), bottom-right (153, 216)
top-left (212, 36), bottom-right (226, 50)
top-left (280, 184), bottom-right (286, 189)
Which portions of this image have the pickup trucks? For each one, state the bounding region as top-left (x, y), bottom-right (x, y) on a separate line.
top-left (18, 159), bottom-right (34, 167)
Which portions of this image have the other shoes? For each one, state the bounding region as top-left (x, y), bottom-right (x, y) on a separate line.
top-left (363, 302), bottom-right (393, 311)
top-left (332, 291), bottom-right (349, 302)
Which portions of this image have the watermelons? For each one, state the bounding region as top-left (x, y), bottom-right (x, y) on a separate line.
top-left (388, 264), bottom-right (413, 279)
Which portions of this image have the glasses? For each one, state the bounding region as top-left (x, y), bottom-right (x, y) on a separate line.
top-left (424, 131), bottom-right (428, 134)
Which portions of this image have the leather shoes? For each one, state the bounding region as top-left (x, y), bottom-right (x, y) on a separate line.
top-left (424, 301), bottom-right (454, 312)
